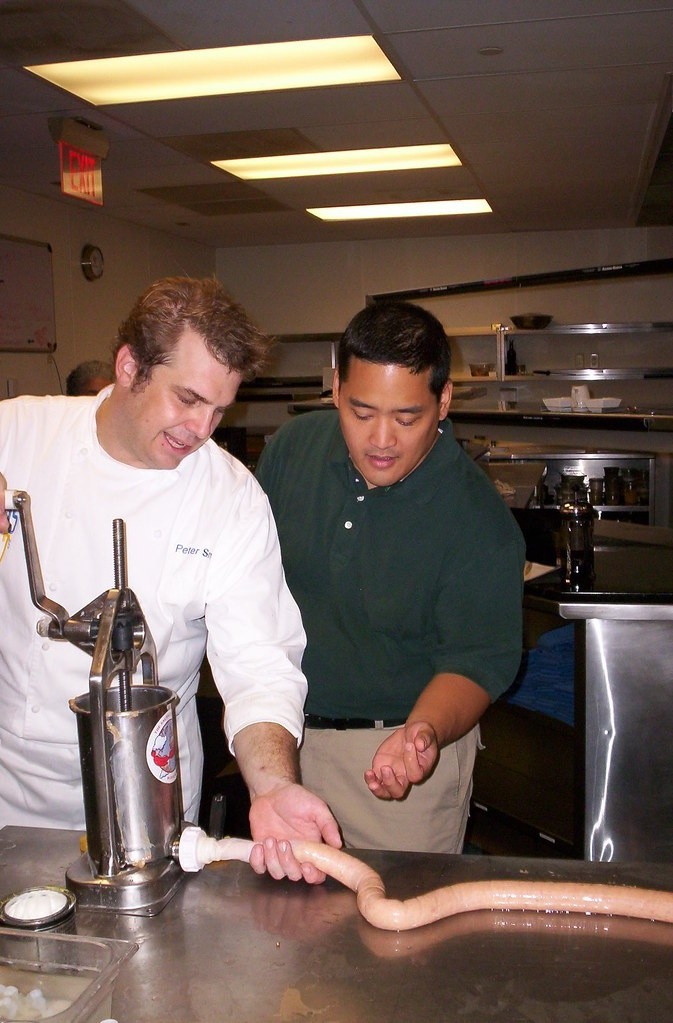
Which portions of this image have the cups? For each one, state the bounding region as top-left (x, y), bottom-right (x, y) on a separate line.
top-left (69, 685), bottom-right (184, 867)
top-left (571, 385), bottom-right (590, 412)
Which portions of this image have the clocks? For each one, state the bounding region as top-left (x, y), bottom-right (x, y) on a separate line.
top-left (81, 245), bottom-right (104, 282)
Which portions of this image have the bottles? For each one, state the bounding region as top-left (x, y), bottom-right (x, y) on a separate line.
top-left (588, 467), bottom-right (650, 506)
top-left (507, 339), bottom-right (516, 375)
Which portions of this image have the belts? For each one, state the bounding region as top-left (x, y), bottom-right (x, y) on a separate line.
top-left (304, 712), bottom-right (376, 731)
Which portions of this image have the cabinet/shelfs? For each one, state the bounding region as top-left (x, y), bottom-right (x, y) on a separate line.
top-left (485, 444), bottom-right (655, 525)
top-left (329, 318), bottom-right (673, 434)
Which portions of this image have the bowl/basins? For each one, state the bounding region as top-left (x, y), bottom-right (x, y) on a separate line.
top-left (543, 397), bottom-right (571, 413)
top-left (510, 314), bottom-right (553, 330)
top-left (469, 363), bottom-right (491, 376)
top-left (585, 398), bottom-right (622, 414)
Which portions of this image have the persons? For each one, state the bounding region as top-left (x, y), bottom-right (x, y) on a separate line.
top-left (0, 277), bottom-right (342, 884)
top-left (257, 299), bottom-right (526, 855)
top-left (66, 360), bottom-right (115, 396)
